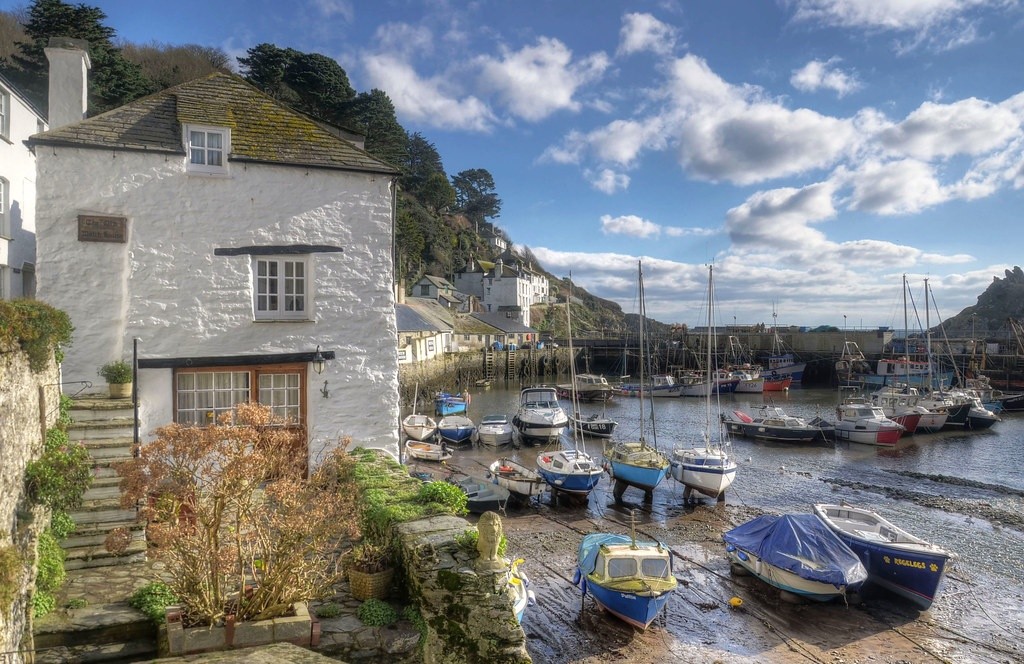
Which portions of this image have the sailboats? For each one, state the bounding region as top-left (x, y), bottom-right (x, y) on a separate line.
top-left (665, 253), bottom-right (737, 503)
top-left (599, 259), bottom-right (672, 504)
top-left (534, 292), bottom-right (605, 505)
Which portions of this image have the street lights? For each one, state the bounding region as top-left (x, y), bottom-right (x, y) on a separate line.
top-left (732, 315), bottom-right (737, 336)
top-left (971, 313), bottom-right (977, 340)
top-left (843, 315), bottom-right (847, 342)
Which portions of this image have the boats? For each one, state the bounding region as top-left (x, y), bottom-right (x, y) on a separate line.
top-left (405, 438), bottom-right (456, 463)
top-left (554, 337), bottom-right (617, 402)
top-left (574, 508), bottom-right (679, 632)
top-left (437, 413), bottom-right (475, 444)
top-left (477, 415), bottom-right (513, 448)
top-left (445, 472), bottom-right (511, 515)
top-left (829, 271), bottom-right (1024, 447)
top-left (605, 296), bottom-right (808, 398)
top-left (488, 456), bottom-right (548, 503)
top-left (400, 381), bottom-right (439, 442)
top-left (567, 410), bottom-right (618, 440)
top-left (433, 365), bottom-right (471, 416)
top-left (498, 554), bottom-right (529, 626)
top-left (810, 497), bottom-right (960, 612)
top-left (513, 382), bottom-right (569, 444)
top-left (716, 393), bottom-right (839, 448)
top-left (720, 511), bottom-right (868, 611)
